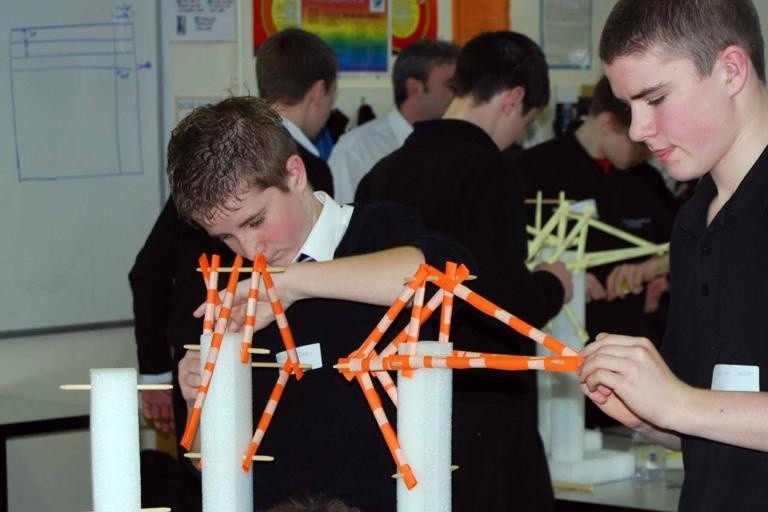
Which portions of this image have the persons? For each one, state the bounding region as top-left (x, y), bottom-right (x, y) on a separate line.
top-left (166, 89), bottom-right (479, 511)
top-left (332, 30), bottom-right (574, 511)
top-left (575, 0), bottom-right (768, 510)
top-left (324, 37), bottom-right (465, 210)
top-left (126, 25), bottom-right (340, 511)
top-left (520, 75), bottom-right (683, 436)
